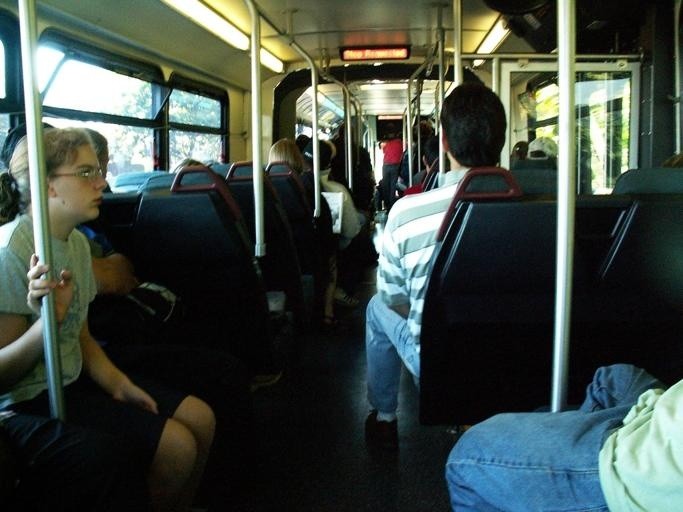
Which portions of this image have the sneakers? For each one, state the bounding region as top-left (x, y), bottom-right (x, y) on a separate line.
top-left (333, 287), bottom-right (359, 305)
top-left (364, 408), bottom-right (397, 449)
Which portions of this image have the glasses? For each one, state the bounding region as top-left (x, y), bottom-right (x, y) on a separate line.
top-left (52, 169), bottom-right (102, 180)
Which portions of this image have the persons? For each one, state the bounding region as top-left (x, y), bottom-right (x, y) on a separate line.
top-left (172, 156), bottom-right (204, 172)
top-left (0, 126), bottom-right (218, 511)
top-left (265, 138), bottom-right (361, 330)
top-left (295, 120), bottom-right (447, 250)
top-left (443, 362), bottom-right (683, 512)
top-left (510, 141), bottom-right (530, 161)
top-left (82, 128), bottom-right (115, 194)
top-left (363, 78), bottom-right (507, 465)
top-left (1, 121), bottom-right (180, 392)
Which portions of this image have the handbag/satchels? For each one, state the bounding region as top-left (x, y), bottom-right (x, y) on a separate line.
top-left (123, 280), bottom-right (184, 327)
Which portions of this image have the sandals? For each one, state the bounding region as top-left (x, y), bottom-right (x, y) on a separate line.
top-left (322, 315), bottom-right (339, 330)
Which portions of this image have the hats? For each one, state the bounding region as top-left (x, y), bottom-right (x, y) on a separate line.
top-left (303, 139), bottom-right (336, 164)
